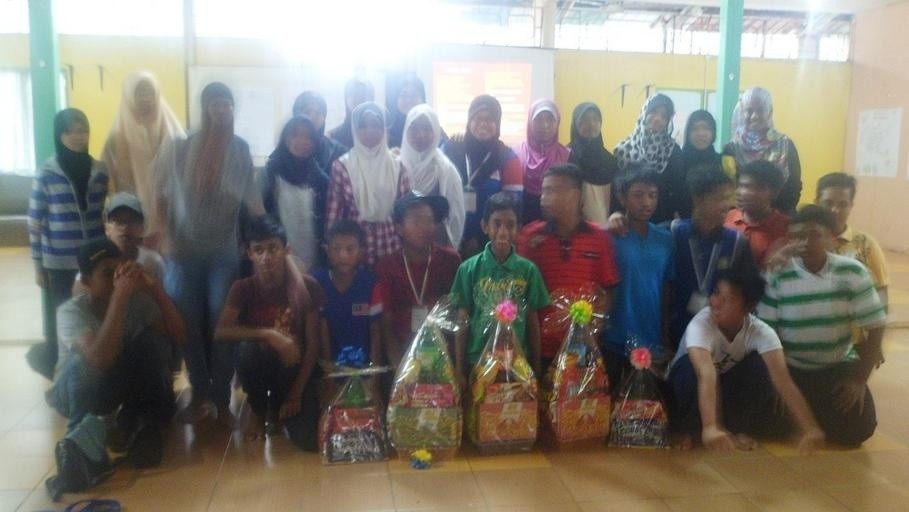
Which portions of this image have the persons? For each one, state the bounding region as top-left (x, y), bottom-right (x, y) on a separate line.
top-left (612, 174), bottom-right (757, 336)
top-left (330, 78), bottom-right (382, 146)
top-left (388, 74), bottom-right (449, 145)
top-left (285, 222), bottom-right (382, 425)
top-left (722, 87), bottom-right (803, 212)
top-left (159, 82), bottom-right (257, 421)
top-left (517, 164), bottom-right (618, 383)
top-left (816, 174), bottom-right (885, 369)
top-left (294, 92), bottom-right (346, 165)
top-left (725, 162), bottom-right (795, 260)
top-left (396, 106), bottom-right (466, 248)
top-left (613, 92), bottom-right (682, 213)
top-left (568, 104), bottom-right (618, 165)
top-left (448, 195), bottom-right (552, 445)
top-left (668, 269), bottom-right (826, 454)
top-left (604, 175), bottom-right (678, 395)
top-left (376, 194), bottom-right (463, 422)
top-left (512, 99), bottom-right (574, 189)
top-left (263, 119), bottom-right (329, 270)
top-left (682, 109), bottom-right (723, 211)
top-left (758, 205), bottom-right (885, 450)
top-left (30, 110), bottom-right (108, 369)
top-left (217, 213), bottom-right (319, 420)
top-left (71, 193), bottom-right (171, 319)
top-left (101, 71), bottom-right (189, 242)
top-left (45, 237), bottom-right (191, 462)
top-left (442, 91), bottom-right (520, 234)
top-left (324, 104), bottom-right (402, 264)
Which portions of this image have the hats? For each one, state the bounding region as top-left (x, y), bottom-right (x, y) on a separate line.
top-left (106, 191), bottom-right (145, 218)
top-left (75, 239), bottom-right (126, 271)
top-left (395, 189), bottom-right (451, 224)
top-left (532, 102), bottom-right (559, 121)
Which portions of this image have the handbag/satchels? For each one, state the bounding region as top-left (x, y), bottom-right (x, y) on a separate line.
top-left (46, 413), bottom-right (127, 503)
